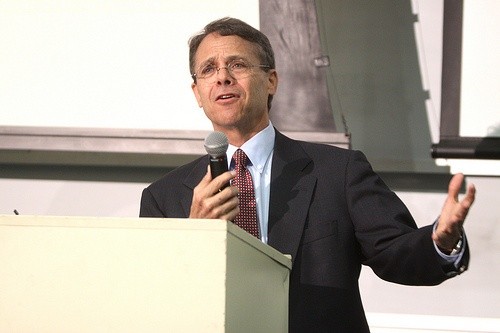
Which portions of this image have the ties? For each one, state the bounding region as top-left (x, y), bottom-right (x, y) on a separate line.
top-left (231, 148), bottom-right (261, 239)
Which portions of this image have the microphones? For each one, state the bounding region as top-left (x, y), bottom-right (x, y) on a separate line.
top-left (203, 133), bottom-right (234, 224)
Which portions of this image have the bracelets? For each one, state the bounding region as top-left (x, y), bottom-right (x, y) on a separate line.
top-left (438, 235), bottom-right (462, 256)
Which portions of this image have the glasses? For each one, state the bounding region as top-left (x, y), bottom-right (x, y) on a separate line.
top-left (193, 58), bottom-right (270, 79)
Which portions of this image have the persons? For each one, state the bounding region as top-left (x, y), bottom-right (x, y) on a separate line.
top-left (139, 17), bottom-right (477, 333)
